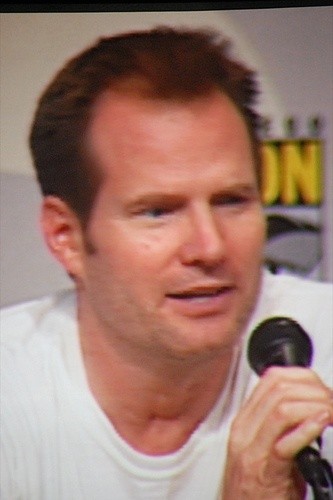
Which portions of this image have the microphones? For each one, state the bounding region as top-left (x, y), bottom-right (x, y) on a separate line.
top-left (246, 316), bottom-right (333, 500)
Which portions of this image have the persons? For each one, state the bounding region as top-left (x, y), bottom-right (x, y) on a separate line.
top-left (0, 25), bottom-right (333, 500)
